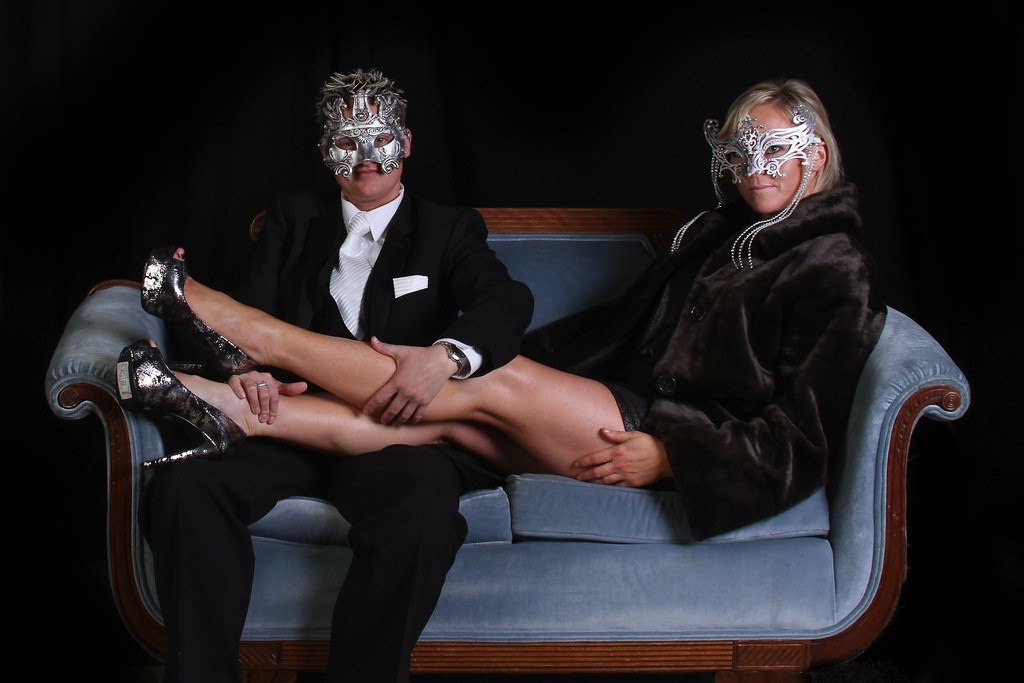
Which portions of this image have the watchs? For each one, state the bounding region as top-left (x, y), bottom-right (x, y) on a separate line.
top-left (438, 341), bottom-right (469, 376)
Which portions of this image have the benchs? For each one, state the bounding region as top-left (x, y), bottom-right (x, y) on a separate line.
top-left (46, 207), bottom-right (969, 683)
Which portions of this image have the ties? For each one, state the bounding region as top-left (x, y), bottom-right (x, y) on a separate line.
top-left (329, 213), bottom-right (372, 341)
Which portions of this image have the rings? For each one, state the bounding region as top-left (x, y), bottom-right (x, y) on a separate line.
top-left (256, 382), bottom-right (266, 387)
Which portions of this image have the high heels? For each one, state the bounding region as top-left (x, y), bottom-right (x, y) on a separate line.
top-left (115, 338), bottom-right (248, 471)
top-left (141, 248), bottom-right (267, 383)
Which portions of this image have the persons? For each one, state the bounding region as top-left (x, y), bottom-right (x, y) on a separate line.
top-left (137, 69), bottom-right (535, 683)
top-left (117, 82), bottom-right (887, 540)
